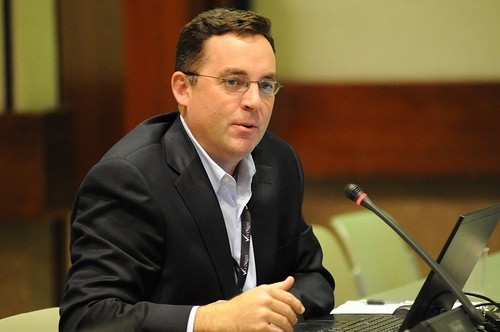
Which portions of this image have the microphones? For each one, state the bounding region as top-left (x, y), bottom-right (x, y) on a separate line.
top-left (345, 182), bottom-right (500, 332)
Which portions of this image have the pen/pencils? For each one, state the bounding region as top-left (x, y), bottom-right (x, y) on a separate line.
top-left (368, 300), bottom-right (402, 304)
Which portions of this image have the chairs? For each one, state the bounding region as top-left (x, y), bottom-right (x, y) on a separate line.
top-left (313, 223), bottom-right (363, 308)
top-left (331, 210), bottom-right (421, 295)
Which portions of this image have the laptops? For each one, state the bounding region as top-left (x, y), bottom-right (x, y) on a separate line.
top-left (284, 204), bottom-right (500, 332)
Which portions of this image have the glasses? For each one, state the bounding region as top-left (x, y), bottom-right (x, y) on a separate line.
top-left (184, 68), bottom-right (283, 95)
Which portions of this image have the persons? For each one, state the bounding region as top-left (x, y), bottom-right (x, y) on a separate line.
top-left (58, 6), bottom-right (335, 332)
